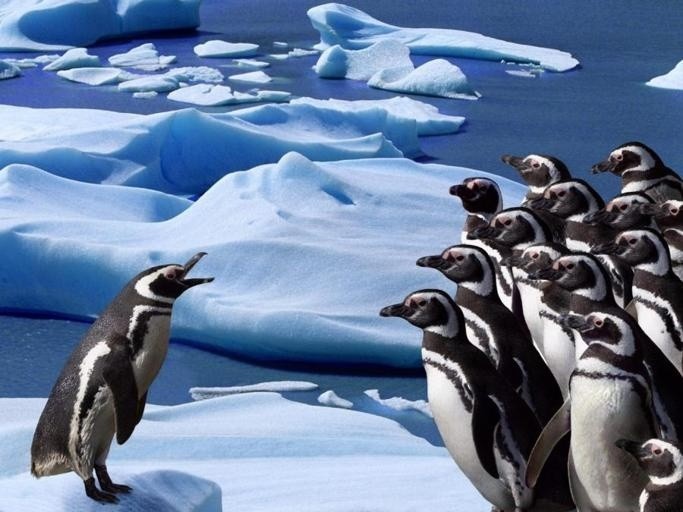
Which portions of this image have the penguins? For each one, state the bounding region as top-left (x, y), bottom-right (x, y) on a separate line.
top-left (30, 251), bottom-right (215, 505)
top-left (379, 140), bottom-right (683, 512)
